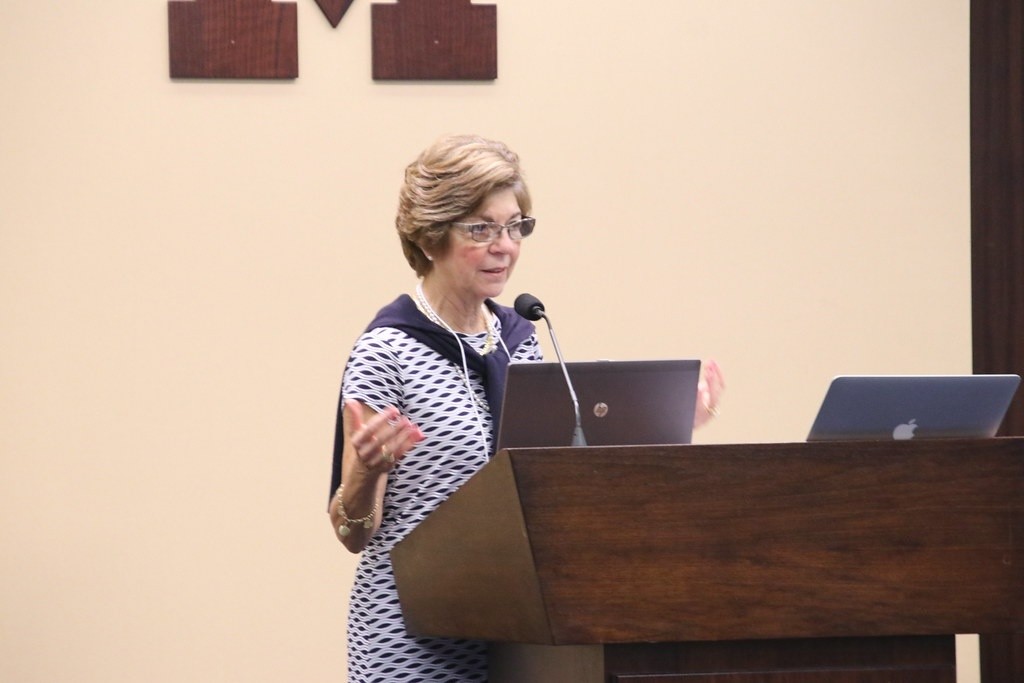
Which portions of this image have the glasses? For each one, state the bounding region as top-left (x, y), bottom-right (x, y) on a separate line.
top-left (447, 215), bottom-right (536, 243)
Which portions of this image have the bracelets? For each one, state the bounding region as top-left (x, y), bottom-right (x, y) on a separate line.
top-left (335, 484), bottom-right (379, 536)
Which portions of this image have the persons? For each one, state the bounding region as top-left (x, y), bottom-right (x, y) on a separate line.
top-left (328, 135), bottom-right (724, 683)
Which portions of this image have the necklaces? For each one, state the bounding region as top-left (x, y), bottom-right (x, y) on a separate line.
top-left (414, 283), bottom-right (494, 412)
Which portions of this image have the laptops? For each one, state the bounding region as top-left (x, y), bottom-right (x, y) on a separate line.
top-left (496, 359), bottom-right (701, 445)
top-left (807, 374), bottom-right (1022, 442)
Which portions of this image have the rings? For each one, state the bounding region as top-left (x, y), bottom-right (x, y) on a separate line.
top-left (380, 446), bottom-right (397, 464)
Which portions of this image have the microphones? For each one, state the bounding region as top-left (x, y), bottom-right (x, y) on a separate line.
top-left (515, 294), bottom-right (587, 446)
